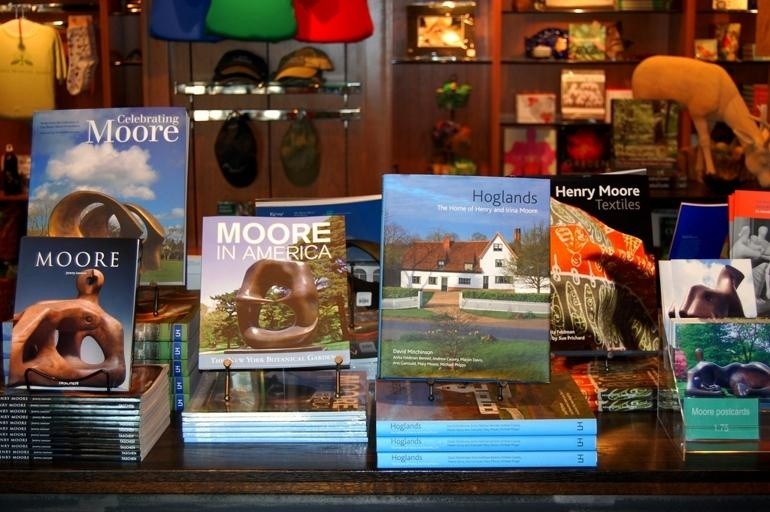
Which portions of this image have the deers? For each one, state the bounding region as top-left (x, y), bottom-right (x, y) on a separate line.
top-left (631, 53), bottom-right (770, 191)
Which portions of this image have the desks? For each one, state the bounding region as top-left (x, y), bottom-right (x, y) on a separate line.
top-left (0, 404), bottom-right (770, 512)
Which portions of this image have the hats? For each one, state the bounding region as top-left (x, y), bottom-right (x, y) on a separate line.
top-left (275, 46), bottom-right (335, 80)
top-left (280, 116), bottom-right (320, 186)
top-left (210, 50), bottom-right (269, 85)
top-left (214, 116), bottom-right (257, 186)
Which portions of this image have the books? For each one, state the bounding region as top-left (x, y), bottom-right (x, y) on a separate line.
top-left (25, 107), bottom-right (190, 285)
top-left (504, 71), bottom-right (678, 186)
top-left (0, 238), bottom-right (201, 470)
top-left (742, 83), bottom-right (769, 129)
top-left (181, 169), bottom-right (770, 472)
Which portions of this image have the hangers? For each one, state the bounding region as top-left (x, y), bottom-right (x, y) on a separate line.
top-left (11, 0), bottom-right (27, 19)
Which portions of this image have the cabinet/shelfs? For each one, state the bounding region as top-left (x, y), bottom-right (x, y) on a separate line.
top-left (388, 0), bottom-right (770, 66)
